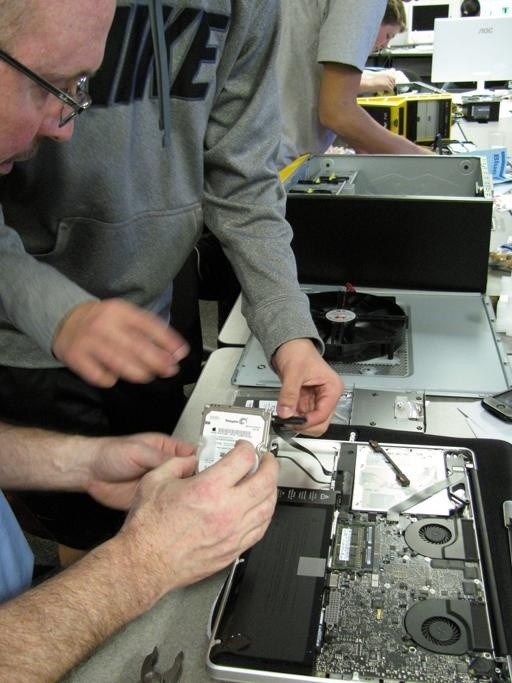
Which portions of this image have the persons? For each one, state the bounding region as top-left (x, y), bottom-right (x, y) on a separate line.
top-left (357, 0), bottom-right (409, 95)
top-left (0, 0), bottom-right (280, 683)
top-left (279, 0), bottom-right (435, 177)
top-left (0, 0), bottom-right (344, 554)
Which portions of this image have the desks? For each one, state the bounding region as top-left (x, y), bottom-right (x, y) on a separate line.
top-left (215, 282), bottom-right (512, 398)
top-left (64, 344), bottom-right (511, 681)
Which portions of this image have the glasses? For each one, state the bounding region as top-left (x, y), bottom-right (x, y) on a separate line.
top-left (0, 49), bottom-right (94, 127)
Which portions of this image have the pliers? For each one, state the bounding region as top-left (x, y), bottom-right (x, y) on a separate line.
top-left (140, 645), bottom-right (184, 683)
